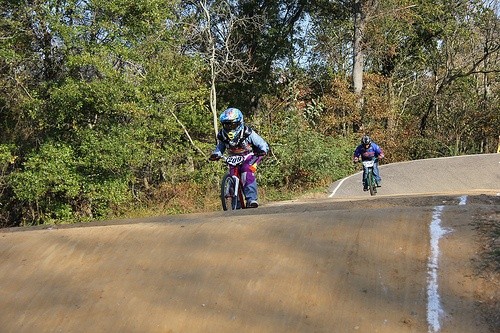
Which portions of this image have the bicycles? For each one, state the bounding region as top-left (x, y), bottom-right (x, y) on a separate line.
top-left (354, 156), bottom-right (381, 195)
top-left (213, 152), bottom-right (249, 210)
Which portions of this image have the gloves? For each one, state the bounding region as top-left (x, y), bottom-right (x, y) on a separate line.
top-left (254, 150), bottom-right (264, 158)
top-left (209, 154), bottom-right (220, 162)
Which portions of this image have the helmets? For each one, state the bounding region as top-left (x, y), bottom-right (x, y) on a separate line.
top-left (361, 136), bottom-right (371, 149)
top-left (220, 108), bottom-right (243, 140)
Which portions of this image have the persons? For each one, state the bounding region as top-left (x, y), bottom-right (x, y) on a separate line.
top-left (354, 136), bottom-right (384, 191)
top-left (210, 108), bottom-right (269, 210)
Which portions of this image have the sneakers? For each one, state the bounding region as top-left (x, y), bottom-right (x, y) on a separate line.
top-left (376, 180), bottom-right (381, 187)
top-left (364, 185), bottom-right (369, 191)
top-left (246, 200), bottom-right (258, 208)
